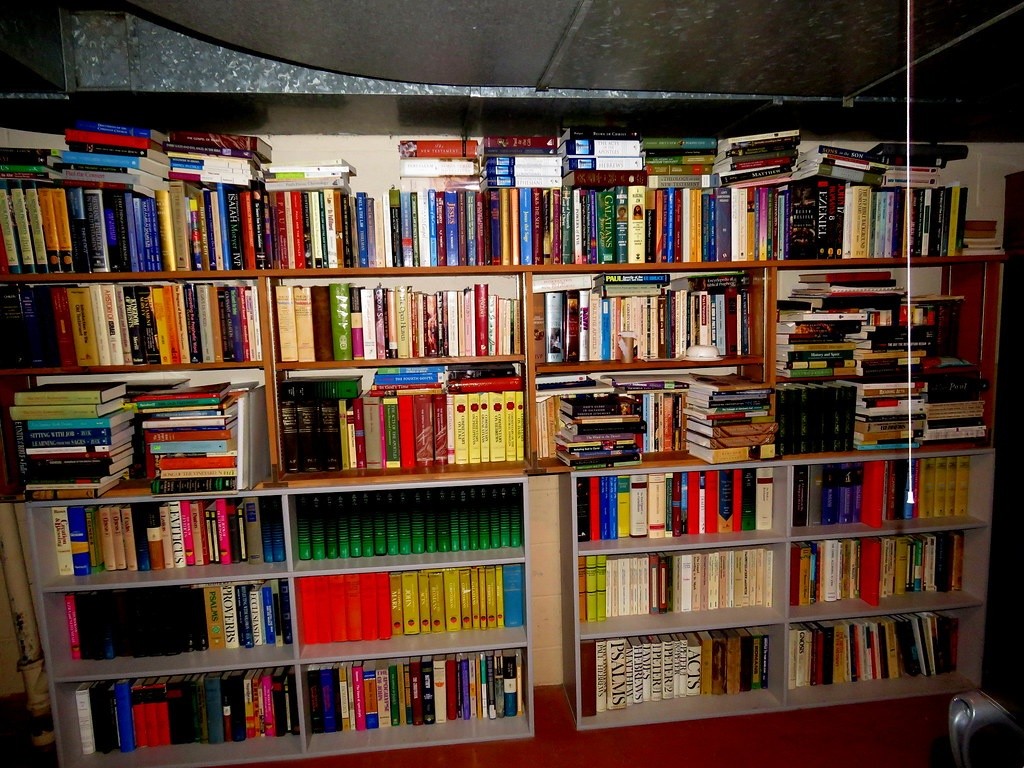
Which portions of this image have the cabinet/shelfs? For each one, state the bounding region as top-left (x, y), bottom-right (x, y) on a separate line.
top-left (557, 448), bottom-right (999, 733)
top-left (0, 253), bottom-right (1013, 504)
top-left (29, 475), bottom-right (537, 768)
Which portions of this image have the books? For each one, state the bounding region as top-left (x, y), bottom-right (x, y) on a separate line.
top-left (0, 119), bottom-right (1002, 756)
